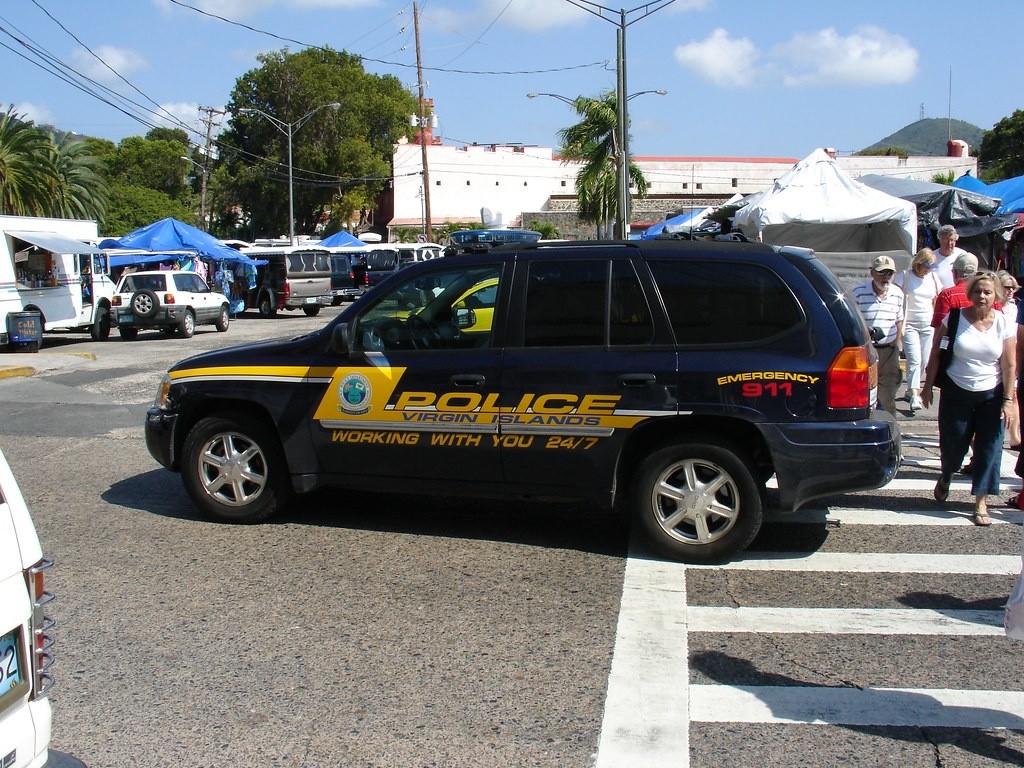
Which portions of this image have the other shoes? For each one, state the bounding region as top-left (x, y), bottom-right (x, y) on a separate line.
top-left (909, 395), bottom-right (923, 410)
top-left (903, 388), bottom-right (911, 402)
top-left (961, 457), bottom-right (973, 475)
top-left (1009, 495), bottom-right (1024, 510)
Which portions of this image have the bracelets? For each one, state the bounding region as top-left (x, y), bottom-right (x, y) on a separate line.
top-left (925, 382), bottom-right (932, 386)
top-left (1003, 398), bottom-right (1013, 401)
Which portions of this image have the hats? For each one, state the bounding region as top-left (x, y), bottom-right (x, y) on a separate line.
top-left (871, 255), bottom-right (896, 273)
top-left (952, 252), bottom-right (979, 272)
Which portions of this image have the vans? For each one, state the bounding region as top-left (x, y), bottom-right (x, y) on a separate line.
top-left (214, 239), bottom-right (446, 320)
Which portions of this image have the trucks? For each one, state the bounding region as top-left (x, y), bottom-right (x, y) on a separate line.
top-left (0, 214), bottom-right (116, 351)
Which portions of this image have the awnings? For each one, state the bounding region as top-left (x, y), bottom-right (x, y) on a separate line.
top-left (3, 230), bottom-right (104, 255)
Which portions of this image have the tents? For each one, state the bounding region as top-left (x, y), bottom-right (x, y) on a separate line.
top-left (640, 148), bottom-right (1024, 255)
top-left (315, 231), bottom-right (368, 246)
top-left (96, 217), bottom-right (268, 269)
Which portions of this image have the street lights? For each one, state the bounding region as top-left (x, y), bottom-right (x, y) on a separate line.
top-left (407, 111), bottom-right (439, 241)
top-left (238, 102), bottom-right (343, 246)
top-left (524, 89), bottom-right (666, 241)
top-left (180, 156), bottom-right (208, 223)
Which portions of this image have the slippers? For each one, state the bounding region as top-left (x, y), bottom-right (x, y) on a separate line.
top-left (934, 476), bottom-right (949, 502)
top-left (973, 507), bottom-right (992, 526)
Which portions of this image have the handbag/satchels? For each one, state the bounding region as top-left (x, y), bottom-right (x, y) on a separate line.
top-left (899, 269), bottom-right (909, 337)
top-left (924, 308), bottom-right (960, 388)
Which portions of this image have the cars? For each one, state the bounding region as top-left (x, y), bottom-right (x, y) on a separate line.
top-left (0, 445), bottom-right (57, 768)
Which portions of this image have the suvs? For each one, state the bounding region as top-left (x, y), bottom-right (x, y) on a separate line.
top-left (110, 270), bottom-right (233, 341)
top-left (143, 228), bottom-right (905, 565)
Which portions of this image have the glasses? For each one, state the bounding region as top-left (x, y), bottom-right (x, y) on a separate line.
top-left (973, 271), bottom-right (995, 277)
top-left (922, 261), bottom-right (938, 268)
top-left (1001, 286), bottom-right (1016, 290)
top-left (877, 271), bottom-right (894, 276)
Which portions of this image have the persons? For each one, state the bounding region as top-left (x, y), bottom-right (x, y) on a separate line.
top-left (932, 225), bottom-right (966, 287)
top-left (920, 271), bottom-right (1017, 526)
top-left (852, 255), bottom-right (905, 462)
top-left (894, 248), bottom-right (943, 410)
top-left (930, 251), bottom-right (1024, 509)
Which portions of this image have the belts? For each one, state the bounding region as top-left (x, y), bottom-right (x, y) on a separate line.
top-left (874, 344), bottom-right (891, 348)
